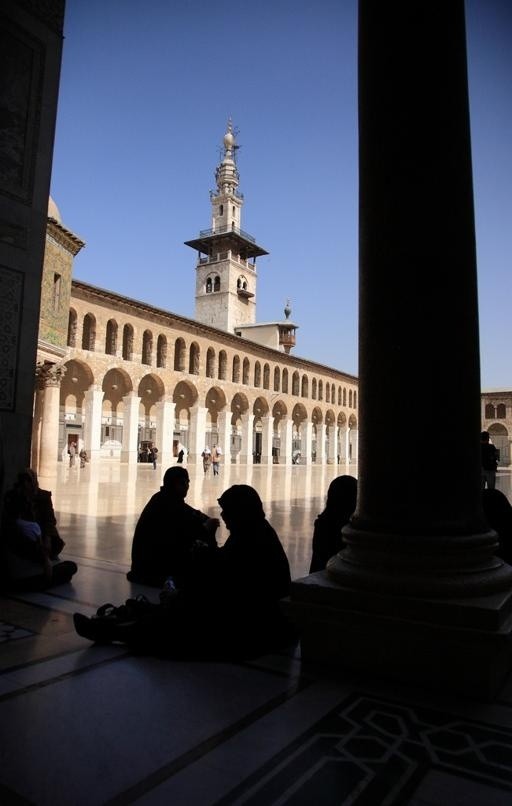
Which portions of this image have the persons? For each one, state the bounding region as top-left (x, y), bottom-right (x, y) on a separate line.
top-left (0, 467), bottom-right (66, 564)
top-left (308, 475), bottom-right (357, 575)
top-left (69, 442), bottom-right (76, 469)
top-left (479, 430), bottom-right (497, 490)
top-left (483, 488), bottom-right (512, 567)
top-left (137, 446), bottom-right (190, 471)
top-left (126, 465), bottom-right (220, 589)
top-left (0, 489), bottom-right (76, 593)
top-left (78, 449), bottom-right (88, 469)
top-left (72, 484), bottom-right (293, 666)
top-left (200, 444), bottom-right (212, 477)
top-left (210, 443), bottom-right (221, 478)
top-left (255, 446), bottom-right (340, 465)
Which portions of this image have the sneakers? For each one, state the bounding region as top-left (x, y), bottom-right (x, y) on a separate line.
top-left (73, 612), bottom-right (113, 644)
